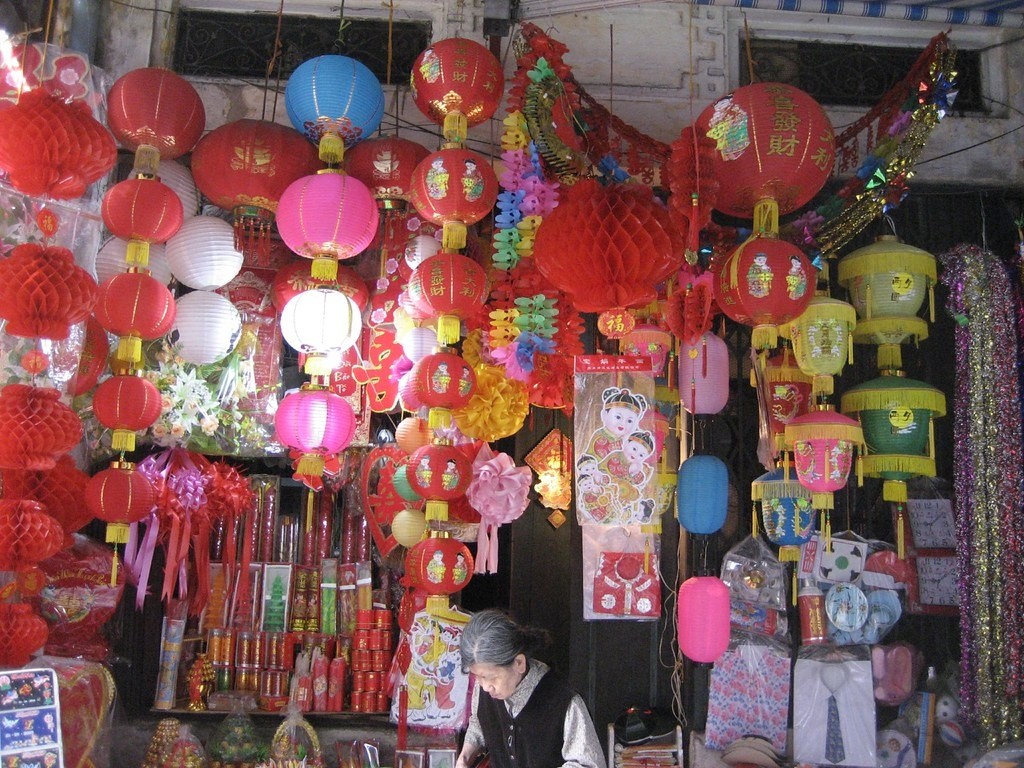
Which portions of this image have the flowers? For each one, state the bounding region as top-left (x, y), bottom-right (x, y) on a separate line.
top-left (136, 361), bottom-right (244, 455)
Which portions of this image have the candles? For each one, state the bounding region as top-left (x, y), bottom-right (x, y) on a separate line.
top-left (398, 685), bottom-right (409, 750)
top-left (179, 477), bottom-right (394, 712)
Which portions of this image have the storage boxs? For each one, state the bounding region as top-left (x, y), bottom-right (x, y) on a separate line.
top-left (794, 644), bottom-right (877, 768)
top-left (227, 562), bottom-right (262, 631)
top-left (704, 644), bottom-right (793, 754)
top-left (287, 562), bottom-right (321, 632)
top-left (394, 746), bottom-right (426, 768)
top-left (198, 560), bottom-right (230, 629)
top-left (815, 529), bottom-right (868, 588)
top-left (424, 742), bottom-right (459, 768)
top-left (898, 691), bottom-right (935, 765)
top-left (259, 561), bottom-right (295, 632)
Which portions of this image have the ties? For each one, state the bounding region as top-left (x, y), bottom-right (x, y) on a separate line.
top-left (825, 696), bottom-right (846, 764)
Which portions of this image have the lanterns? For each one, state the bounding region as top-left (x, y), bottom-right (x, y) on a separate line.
top-left (88, 66), bottom-right (206, 588)
top-left (407, 38), bottom-right (503, 663)
top-left (0, 40), bottom-right (116, 669)
top-left (533, 76), bottom-right (945, 668)
top-left (192, 56), bottom-right (431, 531)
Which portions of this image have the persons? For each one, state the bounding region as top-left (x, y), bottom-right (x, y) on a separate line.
top-left (455, 609), bottom-right (607, 768)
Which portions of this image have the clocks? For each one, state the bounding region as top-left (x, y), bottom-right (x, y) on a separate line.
top-left (891, 496), bottom-right (956, 554)
top-left (905, 553), bottom-right (959, 617)
